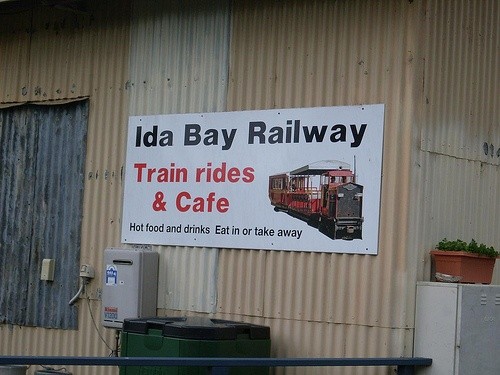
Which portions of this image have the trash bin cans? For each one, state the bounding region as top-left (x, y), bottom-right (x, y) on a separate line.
top-left (116, 316), bottom-right (273, 375)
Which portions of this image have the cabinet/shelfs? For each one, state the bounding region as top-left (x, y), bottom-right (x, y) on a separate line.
top-left (414, 282), bottom-right (499, 374)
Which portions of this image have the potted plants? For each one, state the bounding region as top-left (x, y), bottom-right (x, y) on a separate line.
top-left (431, 238), bottom-right (500, 284)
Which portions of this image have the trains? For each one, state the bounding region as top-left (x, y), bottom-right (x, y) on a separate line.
top-left (270, 159), bottom-right (365, 240)
